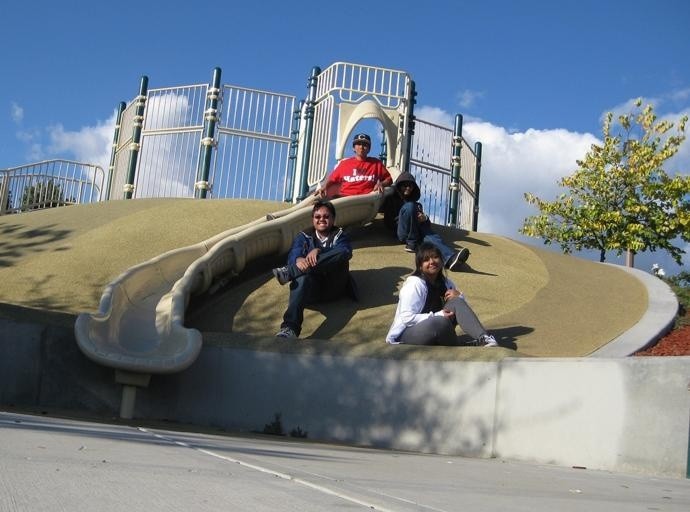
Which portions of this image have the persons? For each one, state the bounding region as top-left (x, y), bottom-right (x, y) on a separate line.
top-left (383, 240), bottom-right (500, 347)
top-left (264, 130), bottom-right (395, 222)
top-left (271, 200), bottom-right (360, 340)
top-left (379, 169), bottom-right (470, 271)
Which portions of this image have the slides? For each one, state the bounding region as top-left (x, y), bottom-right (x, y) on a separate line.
top-left (74, 158), bottom-right (402, 374)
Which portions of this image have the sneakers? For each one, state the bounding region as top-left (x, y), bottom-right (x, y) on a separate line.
top-left (273, 266), bottom-right (289, 285)
top-left (275, 328), bottom-right (297, 338)
top-left (404, 245), bottom-right (415, 252)
top-left (479, 335), bottom-right (498, 347)
top-left (448, 248), bottom-right (469, 271)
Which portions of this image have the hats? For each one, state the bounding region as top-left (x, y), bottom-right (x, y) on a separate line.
top-left (353, 134), bottom-right (371, 145)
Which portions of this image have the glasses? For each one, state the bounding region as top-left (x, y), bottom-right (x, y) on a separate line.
top-left (315, 213), bottom-right (329, 219)
